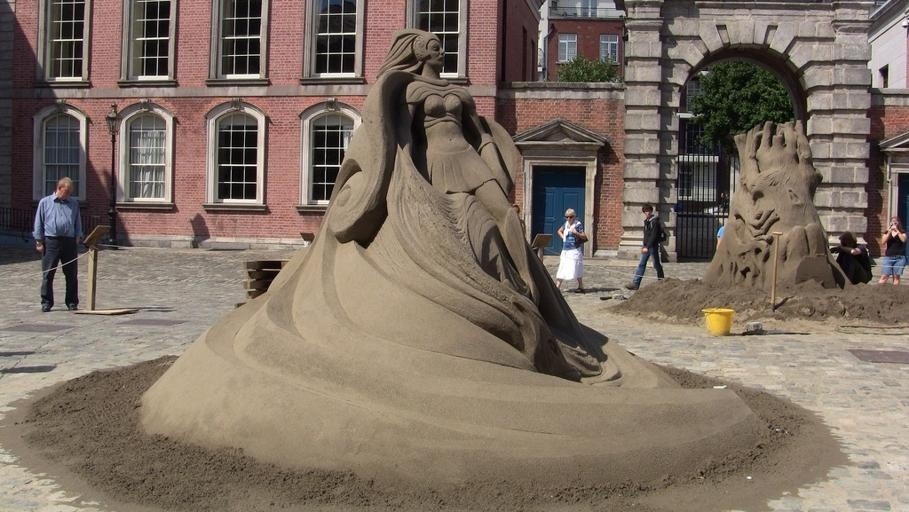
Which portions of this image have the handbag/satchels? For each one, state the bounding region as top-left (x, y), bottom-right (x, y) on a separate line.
top-left (575, 232), bottom-right (589, 246)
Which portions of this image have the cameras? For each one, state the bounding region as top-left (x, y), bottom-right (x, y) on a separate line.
top-left (889, 222), bottom-right (893, 228)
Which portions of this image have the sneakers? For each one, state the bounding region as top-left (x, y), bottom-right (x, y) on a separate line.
top-left (625, 283), bottom-right (639, 290)
top-left (68, 303), bottom-right (78, 310)
top-left (42, 304), bottom-right (50, 312)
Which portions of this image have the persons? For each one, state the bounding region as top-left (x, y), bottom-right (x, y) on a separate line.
top-left (31, 175), bottom-right (85, 312)
top-left (624, 202), bottom-right (664, 289)
top-left (827, 231), bottom-right (873, 286)
top-left (555, 207), bottom-right (588, 293)
top-left (373, 27), bottom-right (544, 310)
top-left (877, 216), bottom-right (908, 286)
top-left (716, 226), bottom-right (724, 250)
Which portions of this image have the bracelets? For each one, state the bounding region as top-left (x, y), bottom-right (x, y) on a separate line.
top-left (36, 240), bottom-right (42, 245)
top-left (897, 231), bottom-right (900, 233)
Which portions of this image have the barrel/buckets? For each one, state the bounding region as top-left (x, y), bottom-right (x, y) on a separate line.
top-left (702, 308), bottom-right (735, 335)
